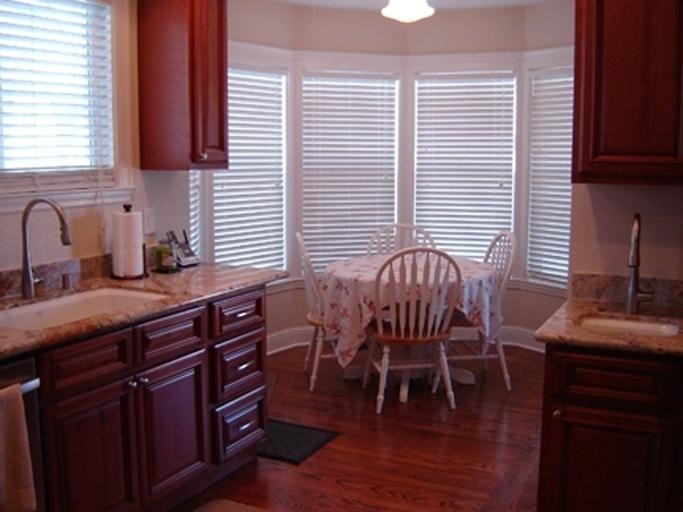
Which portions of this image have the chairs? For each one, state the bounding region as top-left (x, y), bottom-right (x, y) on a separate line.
top-left (367, 223), bottom-right (435, 254)
top-left (362, 247), bottom-right (461, 416)
top-left (430, 232), bottom-right (516, 394)
top-left (295, 231), bottom-right (387, 393)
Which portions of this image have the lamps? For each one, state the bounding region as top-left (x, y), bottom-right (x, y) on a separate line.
top-left (380, 0), bottom-right (434, 25)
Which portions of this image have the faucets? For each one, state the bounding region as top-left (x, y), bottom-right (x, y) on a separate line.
top-left (21, 195), bottom-right (73, 296)
top-left (624, 213), bottom-right (655, 314)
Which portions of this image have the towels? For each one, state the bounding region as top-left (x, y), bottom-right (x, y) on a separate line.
top-left (0, 384), bottom-right (38, 512)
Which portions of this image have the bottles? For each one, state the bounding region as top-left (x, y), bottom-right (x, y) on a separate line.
top-left (156, 240), bottom-right (177, 271)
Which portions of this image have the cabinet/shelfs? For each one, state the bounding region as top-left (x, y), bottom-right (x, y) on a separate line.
top-left (0, 357), bottom-right (46, 512)
top-left (38, 301), bottom-right (210, 512)
top-left (209, 285), bottom-right (268, 483)
top-left (536, 343), bottom-right (683, 512)
top-left (138, 0), bottom-right (228, 171)
top-left (572, 0), bottom-right (683, 183)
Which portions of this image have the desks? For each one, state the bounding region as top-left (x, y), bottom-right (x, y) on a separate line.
top-left (322, 254), bottom-right (504, 403)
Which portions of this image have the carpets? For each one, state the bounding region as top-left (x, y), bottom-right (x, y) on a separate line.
top-left (257, 420), bottom-right (338, 465)
top-left (193, 498), bottom-right (263, 512)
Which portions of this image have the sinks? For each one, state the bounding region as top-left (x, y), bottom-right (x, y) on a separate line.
top-left (574, 309), bottom-right (681, 340)
top-left (0, 281), bottom-right (175, 334)
